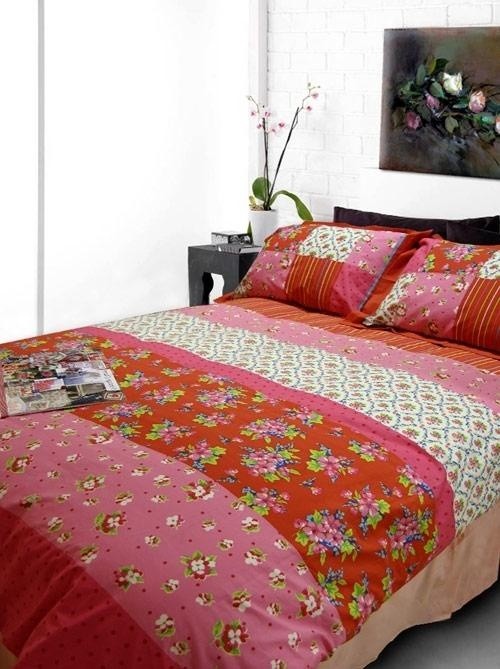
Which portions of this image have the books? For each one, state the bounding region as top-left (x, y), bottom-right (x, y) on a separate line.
top-left (1, 346), bottom-right (127, 421)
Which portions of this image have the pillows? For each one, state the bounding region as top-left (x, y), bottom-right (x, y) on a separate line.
top-left (237, 207), bottom-right (500, 355)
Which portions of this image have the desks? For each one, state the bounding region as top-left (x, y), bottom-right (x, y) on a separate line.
top-left (188, 243), bottom-right (265, 309)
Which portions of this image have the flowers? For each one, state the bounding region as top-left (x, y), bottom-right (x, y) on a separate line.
top-left (391, 54), bottom-right (500, 144)
top-left (245, 81), bottom-right (322, 224)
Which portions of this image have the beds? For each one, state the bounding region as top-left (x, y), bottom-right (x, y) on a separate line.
top-left (0, 291), bottom-right (499, 669)
